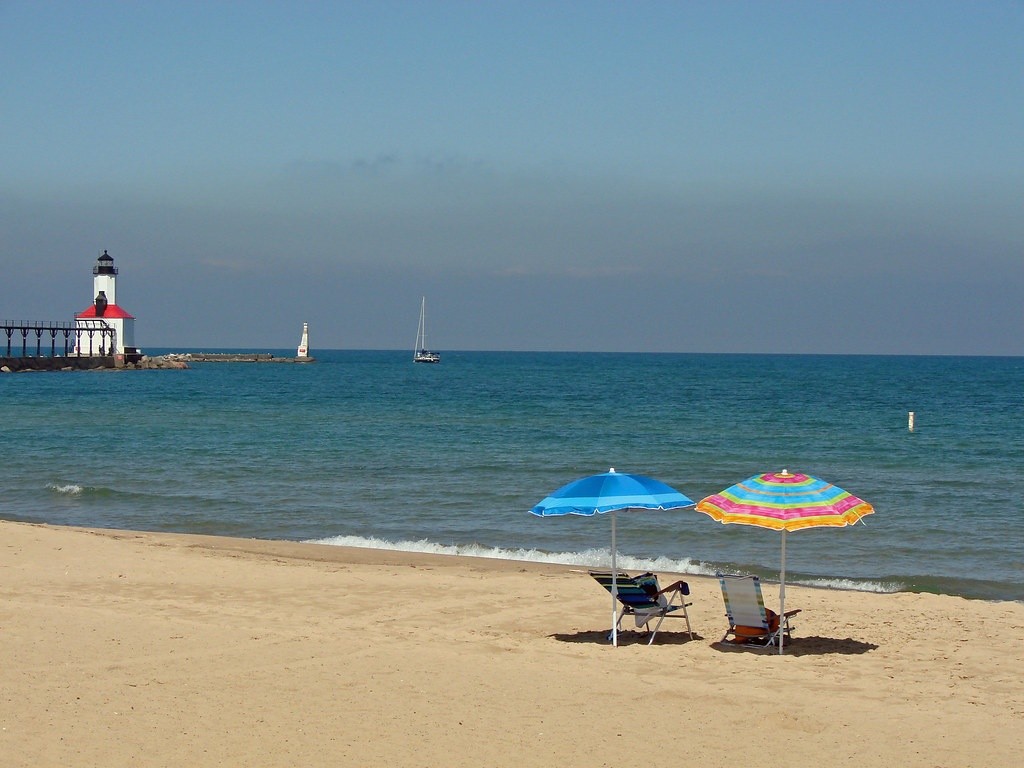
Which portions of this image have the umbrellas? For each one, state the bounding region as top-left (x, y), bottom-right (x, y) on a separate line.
top-left (528, 467), bottom-right (698, 649)
top-left (693, 470), bottom-right (875, 656)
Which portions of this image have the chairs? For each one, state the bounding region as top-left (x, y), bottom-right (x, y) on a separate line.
top-left (586, 570), bottom-right (693, 647)
top-left (715, 571), bottom-right (802, 650)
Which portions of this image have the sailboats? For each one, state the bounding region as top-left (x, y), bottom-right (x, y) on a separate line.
top-left (412, 296), bottom-right (442, 363)
